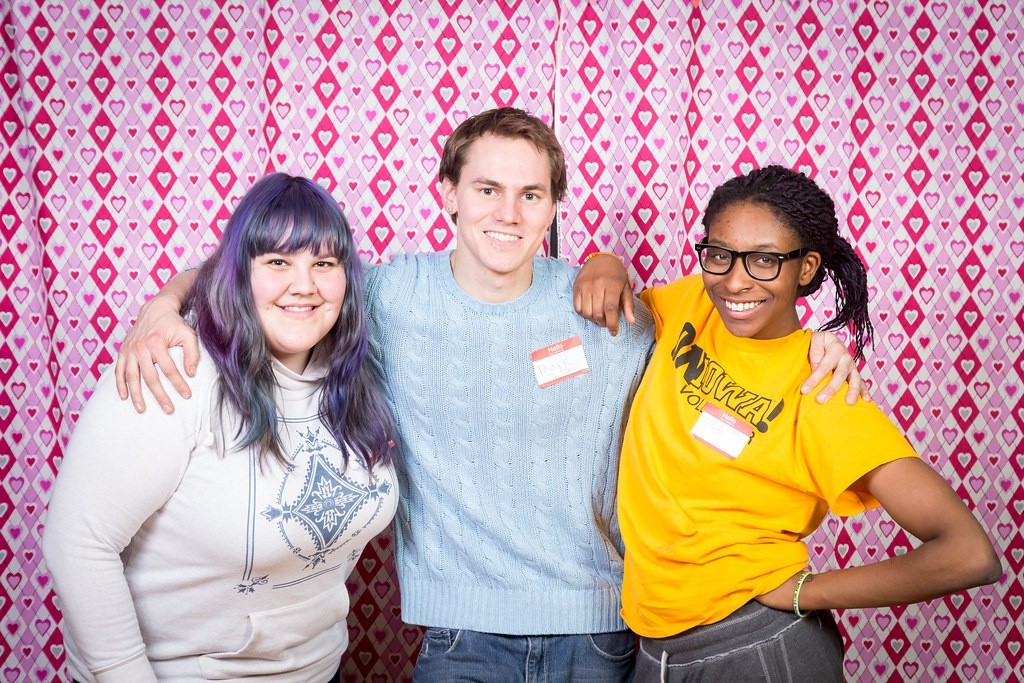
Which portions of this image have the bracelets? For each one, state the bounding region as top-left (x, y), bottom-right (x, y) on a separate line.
top-left (583, 252), bottom-right (629, 284)
top-left (794, 571), bottom-right (816, 618)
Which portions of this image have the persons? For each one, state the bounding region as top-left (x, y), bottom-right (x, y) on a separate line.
top-left (115, 108), bottom-right (875, 683)
top-left (572, 164), bottom-right (1003, 683)
top-left (40, 172), bottom-right (398, 683)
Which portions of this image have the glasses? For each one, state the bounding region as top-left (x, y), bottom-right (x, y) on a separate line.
top-left (695, 236), bottom-right (810, 281)
